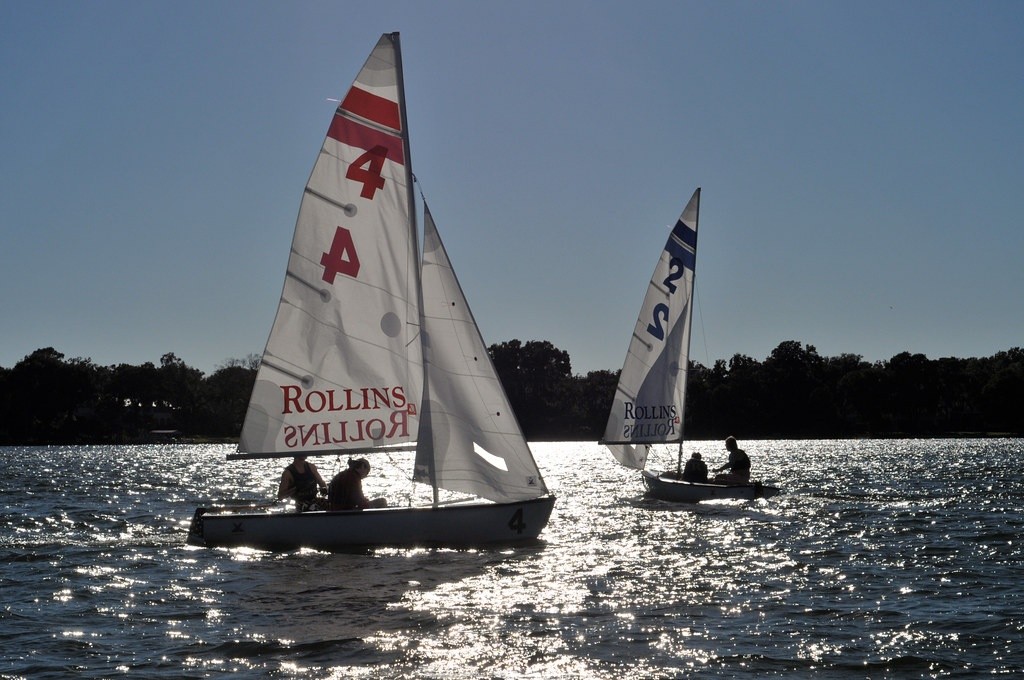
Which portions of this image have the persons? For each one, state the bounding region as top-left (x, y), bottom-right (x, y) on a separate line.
top-left (279, 456), bottom-right (387, 511)
top-left (683, 436), bottom-right (751, 485)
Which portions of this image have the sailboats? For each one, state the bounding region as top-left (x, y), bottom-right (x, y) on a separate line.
top-left (597, 186), bottom-right (775, 496)
top-left (189, 32), bottom-right (556, 544)
